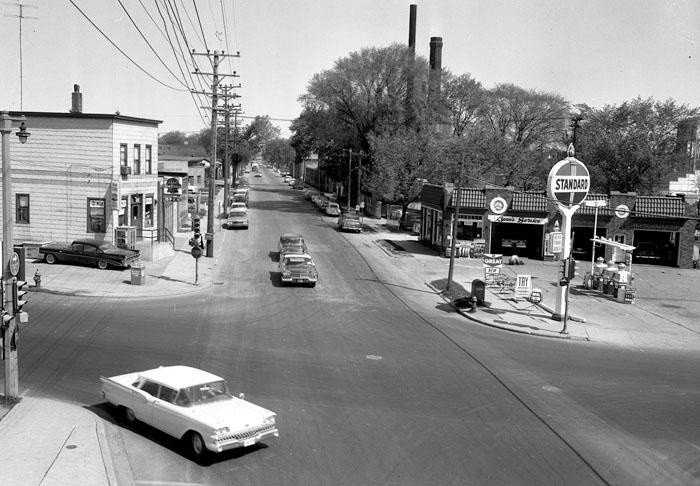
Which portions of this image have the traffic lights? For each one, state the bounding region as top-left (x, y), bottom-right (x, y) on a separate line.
top-left (13, 279), bottom-right (29, 312)
top-left (0, 310), bottom-right (14, 329)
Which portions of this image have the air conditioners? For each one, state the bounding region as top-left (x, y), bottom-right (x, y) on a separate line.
top-left (121, 166), bottom-right (131, 175)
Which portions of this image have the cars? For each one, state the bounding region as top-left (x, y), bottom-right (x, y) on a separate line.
top-left (278, 232), bottom-right (319, 288)
top-left (99, 364), bottom-right (279, 457)
top-left (227, 187), bottom-right (250, 229)
top-left (38, 237), bottom-right (142, 270)
top-left (304, 187), bottom-right (361, 232)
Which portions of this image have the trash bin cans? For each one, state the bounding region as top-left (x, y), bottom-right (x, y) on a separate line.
top-left (472, 279), bottom-right (485, 307)
top-left (130, 262), bottom-right (145, 286)
